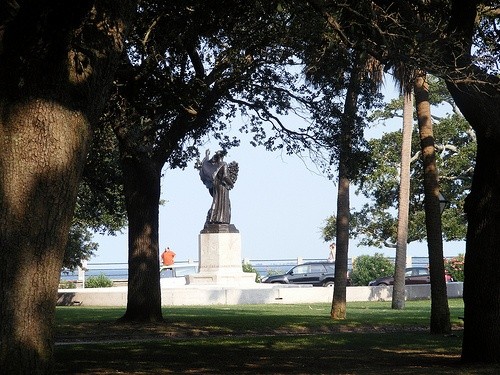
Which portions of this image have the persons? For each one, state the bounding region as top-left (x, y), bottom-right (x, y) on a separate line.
top-left (199, 148), bottom-right (234, 225)
top-left (161, 247), bottom-right (176, 266)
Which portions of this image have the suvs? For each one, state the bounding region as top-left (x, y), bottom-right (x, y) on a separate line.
top-left (159, 265), bottom-right (198, 287)
top-left (260, 261), bottom-right (353, 288)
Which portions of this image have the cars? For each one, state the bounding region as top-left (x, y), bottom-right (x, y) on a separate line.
top-left (368, 267), bottom-right (453, 286)
